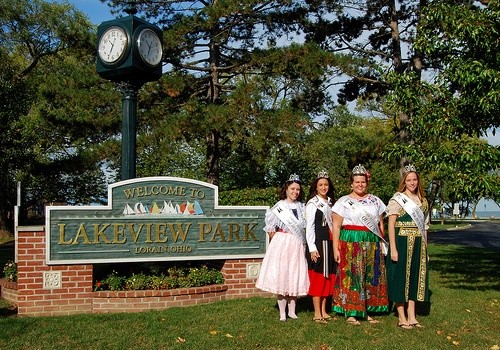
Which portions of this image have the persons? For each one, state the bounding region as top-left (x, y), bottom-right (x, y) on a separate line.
top-left (304, 170), bottom-right (335, 324)
top-left (387, 164), bottom-right (431, 329)
top-left (263, 172), bottom-right (308, 321)
top-left (331, 164), bottom-right (388, 325)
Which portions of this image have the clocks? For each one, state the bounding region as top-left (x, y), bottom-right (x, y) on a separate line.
top-left (137, 28), bottom-right (164, 67)
top-left (97, 24), bottom-right (128, 67)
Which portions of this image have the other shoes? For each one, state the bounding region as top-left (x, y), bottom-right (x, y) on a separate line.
top-left (280, 315), bottom-right (286, 321)
top-left (288, 313), bottom-right (298, 319)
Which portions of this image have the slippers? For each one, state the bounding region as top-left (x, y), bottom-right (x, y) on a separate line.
top-left (312, 317), bottom-right (327, 323)
top-left (397, 321), bottom-right (413, 329)
top-left (346, 316), bottom-right (360, 325)
top-left (407, 321), bottom-right (422, 327)
top-left (322, 315), bottom-right (336, 320)
top-left (365, 317), bottom-right (380, 323)
